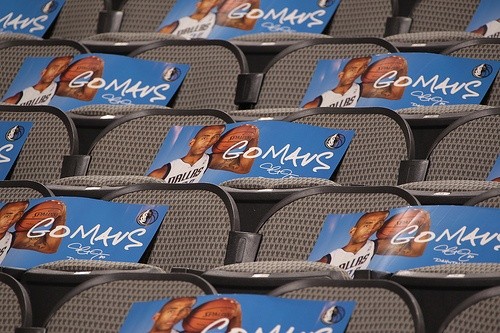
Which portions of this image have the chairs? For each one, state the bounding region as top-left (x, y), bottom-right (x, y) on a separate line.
top-left (0, 0), bottom-right (500, 333)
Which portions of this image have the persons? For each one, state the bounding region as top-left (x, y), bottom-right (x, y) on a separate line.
top-left (147, 124), bottom-right (258, 183)
top-left (471, 19), bottom-right (500, 37)
top-left (148, 296), bottom-right (242, 333)
top-left (318, 211), bottom-right (433, 268)
top-left (2, 56), bottom-right (104, 106)
top-left (158, 0), bottom-right (260, 40)
top-left (302, 57), bottom-right (408, 108)
top-left (0, 199), bottom-right (66, 265)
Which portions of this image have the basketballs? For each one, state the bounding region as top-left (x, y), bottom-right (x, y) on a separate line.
top-left (60, 56), bottom-right (101, 81)
top-left (375, 210), bottom-right (425, 238)
top-left (361, 56), bottom-right (405, 82)
top-left (211, 124), bottom-right (255, 152)
top-left (181, 299), bottom-right (236, 332)
top-left (14, 201), bottom-right (64, 231)
top-left (218, 0), bottom-right (245, 13)
